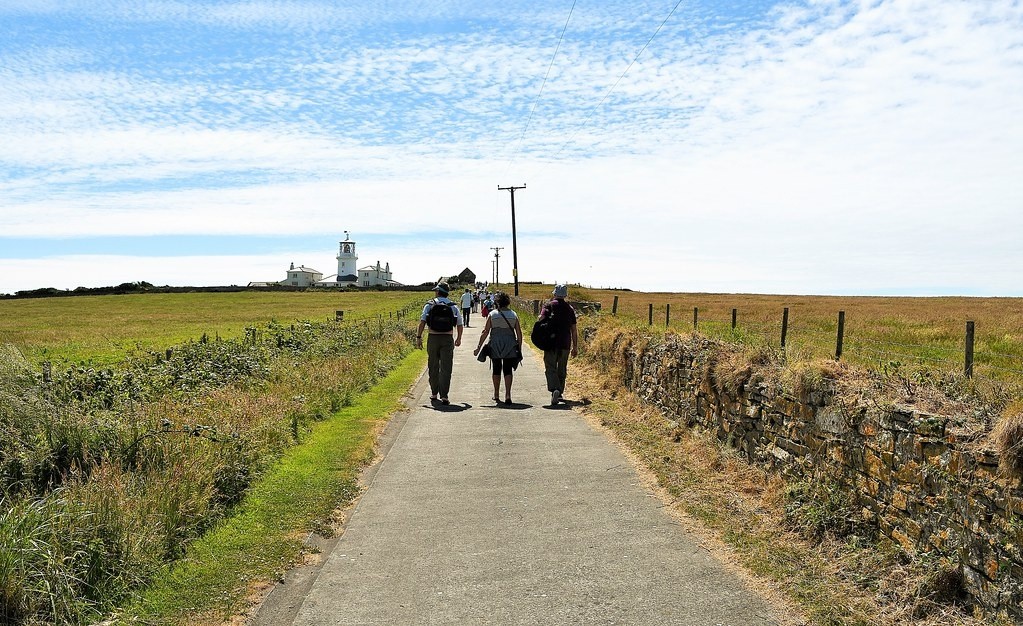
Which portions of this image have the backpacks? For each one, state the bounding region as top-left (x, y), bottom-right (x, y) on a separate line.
top-left (426, 299), bottom-right (458, 332)
top-left (530, 303), bottom-right (562, 350)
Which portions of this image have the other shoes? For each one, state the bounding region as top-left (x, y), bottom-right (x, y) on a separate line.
top-left (464, 322), bottom-right (465, 326)
top-left (492, 397), bottom-right (499, 400)
top-left (551, 390), bottom-right (563, 406)
top-left (430, 395), bottom-right (437, 400)
top-left (467, 325), bottom-right (469, 327)
top-left (505, 399), bottom-right (512, 405)
top-left (443, 400), bottom-right (450, 406)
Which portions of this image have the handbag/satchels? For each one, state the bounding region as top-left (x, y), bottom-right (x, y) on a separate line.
top-left (470, 301), bottom-right (474, 307)
top-left (515, 338), bottom-right (523, 361)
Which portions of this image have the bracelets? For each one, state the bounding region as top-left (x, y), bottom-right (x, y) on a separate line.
top-left (417, 336), bottom-right (422, 339)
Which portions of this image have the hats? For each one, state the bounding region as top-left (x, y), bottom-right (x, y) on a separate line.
top-left (552, 285), bottom-right (568, 296)
top-left (431, 282), bottom-right (450, 294)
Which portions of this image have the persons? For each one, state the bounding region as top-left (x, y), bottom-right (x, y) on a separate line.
top-left (415, 282), bottom-right (463, 402)
top-left (474, 292), bottom-right (523, 403)
top-left (460, 280), bottom-right (500, 327)
top-left (531, 284), bottom-right (578, 405)
